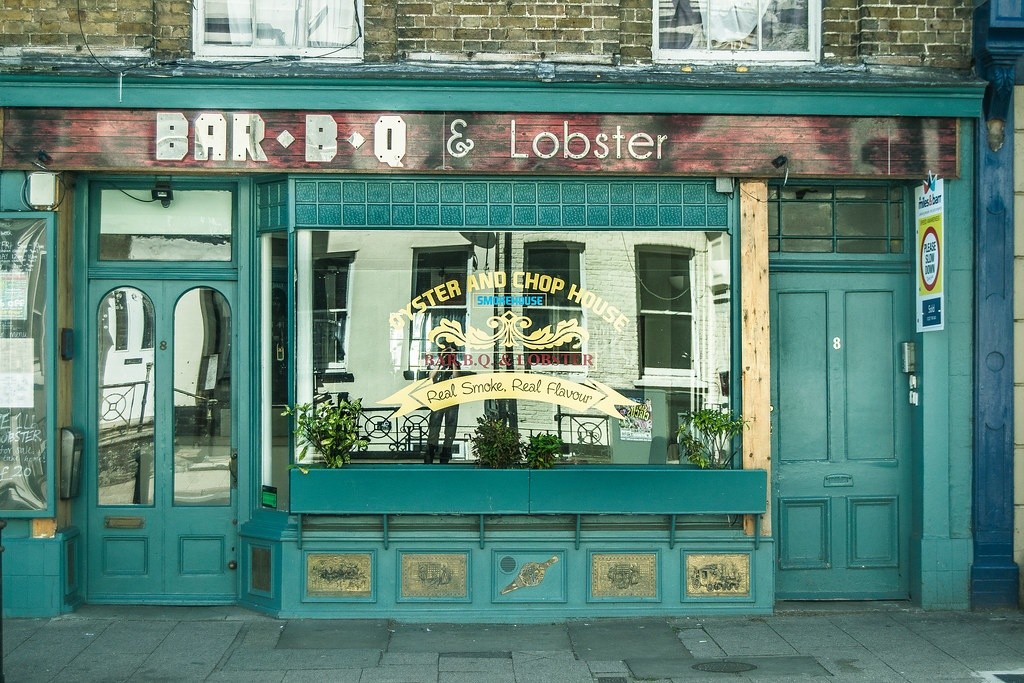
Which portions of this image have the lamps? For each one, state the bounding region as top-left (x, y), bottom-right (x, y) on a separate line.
top-left (150, 181), bottom-right (174, 208)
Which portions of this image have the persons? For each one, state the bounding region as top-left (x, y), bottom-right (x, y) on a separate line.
top-left (423, 340), bottom-right (461, 464)
